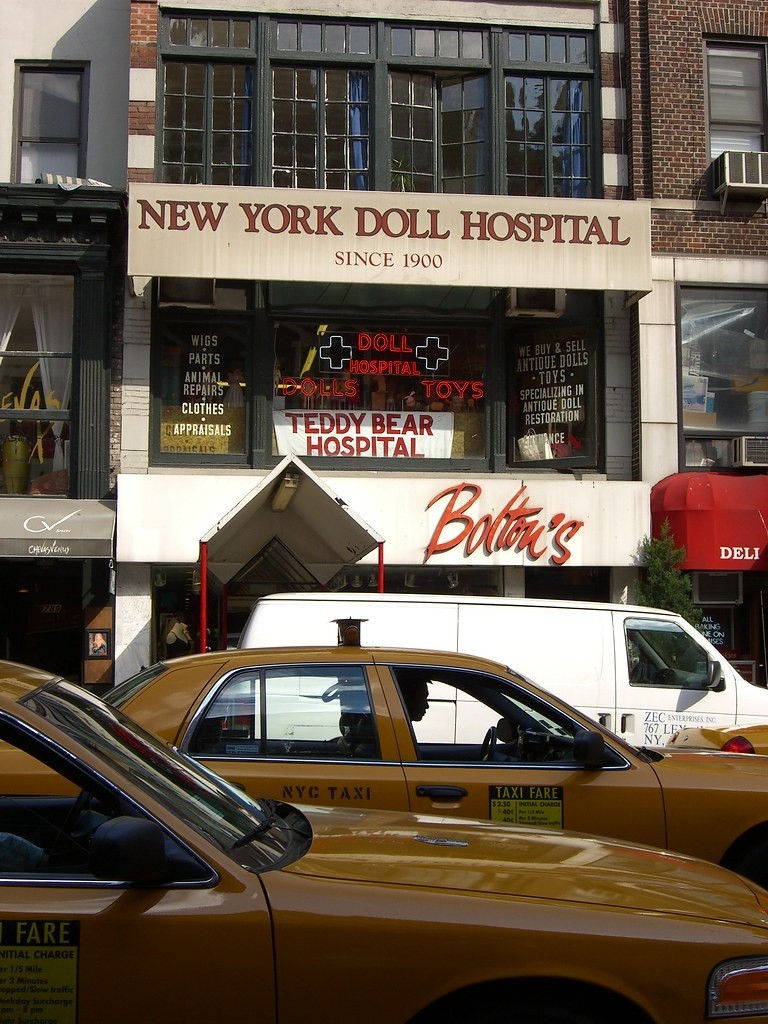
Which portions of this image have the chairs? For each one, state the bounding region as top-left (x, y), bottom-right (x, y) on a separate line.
top-left (338, 707), bottom-right (375, 759)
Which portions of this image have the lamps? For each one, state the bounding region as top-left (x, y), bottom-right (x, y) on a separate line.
top-left (272, 473), bottom-right (299, 512)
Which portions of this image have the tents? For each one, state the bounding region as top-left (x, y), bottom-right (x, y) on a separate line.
top-left (651, 471), bottom-right (768, 571)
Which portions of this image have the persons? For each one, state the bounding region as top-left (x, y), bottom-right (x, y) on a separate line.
top-left (393, 668), bottom-right (434, 721)
top-left (0, 831), bottom-right (50, 871)
top-left (166, 622), bottom-right (194, 659)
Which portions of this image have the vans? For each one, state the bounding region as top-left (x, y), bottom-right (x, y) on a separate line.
top-left (212, 591), bottom-right (768, 772)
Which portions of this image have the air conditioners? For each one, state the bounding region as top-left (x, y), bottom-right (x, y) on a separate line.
top-left (505, 288), bottom-right (567, 319)
top-left (732, 435), bottom-right (768, 468)
top-left (711, 151), bottom-right (768, 199)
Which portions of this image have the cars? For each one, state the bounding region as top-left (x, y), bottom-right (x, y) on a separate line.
top-left (1, 660), bottom-right (768, 1024)
top-left (0, 645), bottom-right (766, 894)
